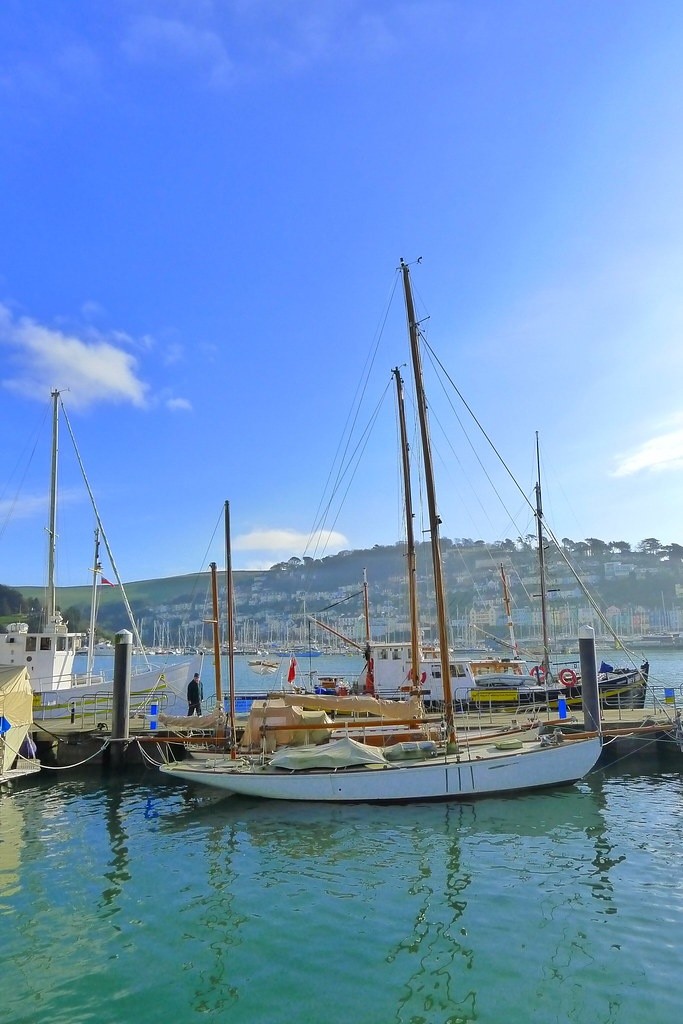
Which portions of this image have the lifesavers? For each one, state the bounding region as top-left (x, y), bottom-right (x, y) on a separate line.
top-left (530, 666), bottom-right (546, 682)
top-left (407, 668), bottom-right (427, 687)
top-left (559, 669), bottom-right (577, 687)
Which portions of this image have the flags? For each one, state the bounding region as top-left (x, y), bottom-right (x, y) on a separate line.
top-left (599, 661), bottom-right (613, 673)
top-left (288, 653), bottom-right (297, 683)
top-left (101, 575), bottom-right (115, 588)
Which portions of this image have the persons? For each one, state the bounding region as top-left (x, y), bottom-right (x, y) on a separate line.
top-left (187, 672), bottom-right (204, 717)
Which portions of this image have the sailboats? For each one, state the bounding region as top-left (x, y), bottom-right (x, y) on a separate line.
top-left (1, 254), bottom-right (651, 803)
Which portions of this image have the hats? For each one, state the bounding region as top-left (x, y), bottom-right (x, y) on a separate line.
top-left (194, 673), bottom-right (199, 677)
